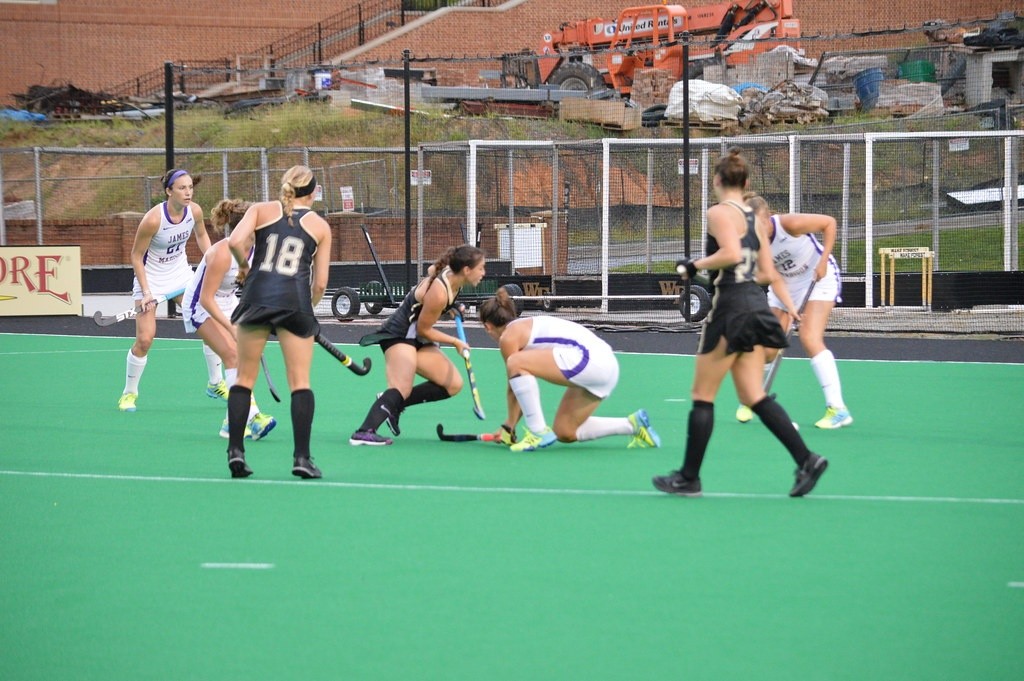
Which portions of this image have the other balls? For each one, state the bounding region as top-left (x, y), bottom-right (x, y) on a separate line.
top-left (736, 409), bottom-right (753, 423)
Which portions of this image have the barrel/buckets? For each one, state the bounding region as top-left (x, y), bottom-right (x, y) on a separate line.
top-left (851, 67), bottom-right (883, 112)
top-left (315, 68), bottom-right (333, 91)
top-left (899, 60), bottom-right (937, 85)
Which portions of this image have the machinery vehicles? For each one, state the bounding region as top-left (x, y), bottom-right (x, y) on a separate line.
top-left (499, 0), bottom-right (801, 106)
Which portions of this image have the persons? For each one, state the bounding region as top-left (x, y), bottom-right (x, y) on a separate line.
top-left (735, 191), bottom-right (854, 429)
top-left (651, 143), bottom-right (828, 497)
top-left (480, 287), bottom-right (661, 452)
top-left (227, 164), bottom-right (332, 478)
top-left (117, 168), bottom-right (229, 411)
top-left (181, 199), bottom-right (277, 442)
top-left (349, 244), bottom-right (486, 444)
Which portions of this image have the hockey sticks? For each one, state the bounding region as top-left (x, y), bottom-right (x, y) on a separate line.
top-left (455, 315), bottom-right (488, 422)
top-left (436, 423), bottom-right (518, 444)
top-left (315, 334), bottom-right (373, 376)
top-left (765, 279), bottom-right (817, 401)
top-left (676, 265), bottom-right (710, 286)
top-left (260, 353), bottom-right (281, 404)
top-left (93, 288), bottom-right (185, 328)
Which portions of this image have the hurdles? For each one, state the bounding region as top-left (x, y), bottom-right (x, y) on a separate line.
top-left (877, 246), bottom-right (940, 312)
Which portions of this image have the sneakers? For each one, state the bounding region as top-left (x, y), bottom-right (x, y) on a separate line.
top-left (790, 453), bottom-right (827, 496)
top-left (651, 470), bottom-right (701, 494)
top-left (349, 429), bottom-right (393, 446)
top-left (292, 455), bottom-right (322, 479)
top-left (248, 414), bottom-right (276, 441)
top-left (510, 425), bottom-right (557, 451)
top-left (815, 406), bottom-right (853, 429)
top-left (206, 380), bottom-right (229, 400)
top-left (626, 409), bottom-right (660, 448)
top-left (118, 394), bottom-right (136, 412)
top-left (377, 391), bottom-right (401, 436)
top-left (219, 419), bottom-right (252, 439)
top-left (228, 447), bottom-right (253, 477)
